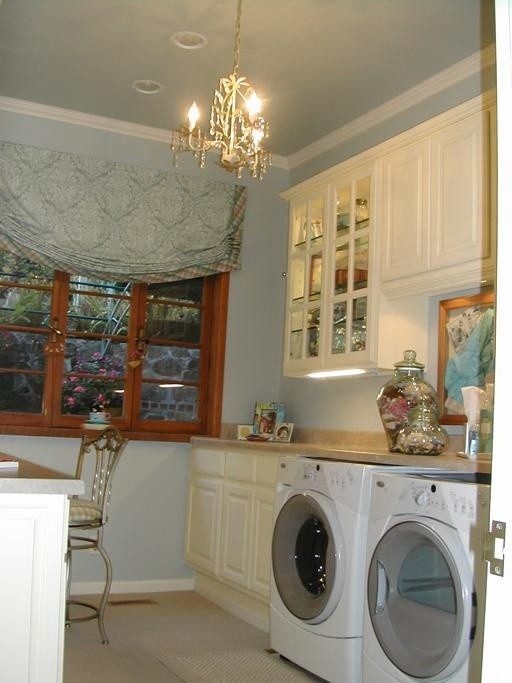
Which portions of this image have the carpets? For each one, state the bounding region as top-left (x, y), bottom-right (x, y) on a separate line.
top-left (157, 646), bottom-right (322, 683)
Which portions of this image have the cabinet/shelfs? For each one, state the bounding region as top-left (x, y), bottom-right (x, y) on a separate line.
top-left (178, 443), bottom-right (282, 601)
top-left (377, 110), bottom-right (496, 301)
top-left (278, 161), bottom-right (429, 380)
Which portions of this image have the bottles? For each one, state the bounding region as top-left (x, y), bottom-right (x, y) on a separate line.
top-left (354, 198), bottom-right (369, 223)
top-left (374, 347), bottom-right (443, 452)
top-left (395, 389), bottom-right (449, 455)
top-left (478, 383), bottom-right (493, 454)
top-left (253, 413), bottom-right (260, 433)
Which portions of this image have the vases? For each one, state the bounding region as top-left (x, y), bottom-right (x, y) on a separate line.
top-left (89, 411), bottom-right (111, 421)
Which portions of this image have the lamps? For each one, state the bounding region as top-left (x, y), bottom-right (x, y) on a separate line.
top-left (171, 0), bottom-right (273, 181)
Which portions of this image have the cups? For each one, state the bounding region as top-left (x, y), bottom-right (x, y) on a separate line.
top-left (464, 422), bottom-right (480, 460)
top-left (335, 314), bottom-right (366, 351)
top-left (89, 411), bottom-right (110, 420)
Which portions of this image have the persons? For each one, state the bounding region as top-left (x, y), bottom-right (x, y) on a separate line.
top-left (259, 412), bottom-right (275, 433)
top-left (277, 426), bottom-right (288, 439)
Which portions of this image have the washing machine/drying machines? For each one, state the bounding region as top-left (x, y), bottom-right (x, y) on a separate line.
top-left (359, 466), bottom-right (491, 682)
top-left (269, 458), bottom-right (409, 682)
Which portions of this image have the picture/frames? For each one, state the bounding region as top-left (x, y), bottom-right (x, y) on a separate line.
top-left (310, 254), bottom-right (321, 295)
top-left (438, 291), bottom-right (496, 425)
top-left (253, 400), bottom-right (286, 439)
top-left (274, 422), bottom-right (294, 443)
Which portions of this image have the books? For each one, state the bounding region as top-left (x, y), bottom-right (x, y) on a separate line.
top-left (0, 454), bottom-right (19, 468)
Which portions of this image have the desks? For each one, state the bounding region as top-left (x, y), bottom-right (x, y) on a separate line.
top-left (0, 451), bottom-right (85, 683)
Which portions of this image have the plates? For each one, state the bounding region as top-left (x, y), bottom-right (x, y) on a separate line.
top-left (246, 433), bottom-right (269, 441)
top-left (84, 420), bottom-right (113, 424)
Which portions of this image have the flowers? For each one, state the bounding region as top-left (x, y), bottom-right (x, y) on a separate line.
top-left (60, 351), bottom-right (124, 412)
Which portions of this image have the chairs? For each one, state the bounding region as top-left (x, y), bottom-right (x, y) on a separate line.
top-left (66, 424), bottom-right (128, 645)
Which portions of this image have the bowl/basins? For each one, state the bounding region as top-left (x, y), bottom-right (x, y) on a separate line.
top-left (309, 308), bottom-right (343, 324)
top-left (337, 269), bottom-right (367, 284)
